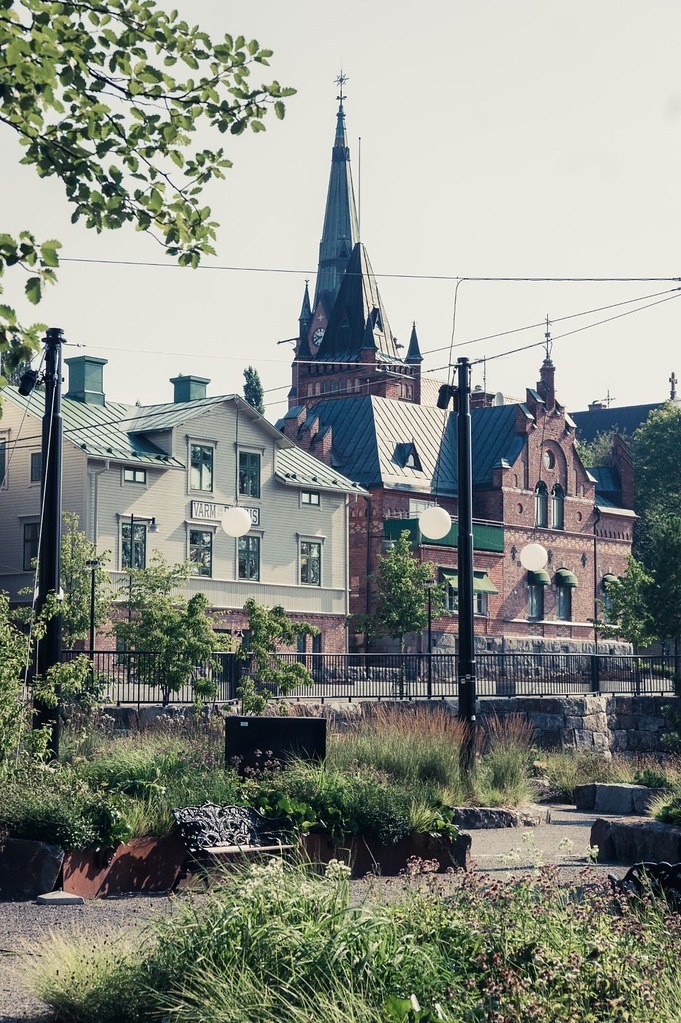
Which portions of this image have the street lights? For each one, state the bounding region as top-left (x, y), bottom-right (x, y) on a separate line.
top-left (17, 325), bottom-right (67, 767)
top-left (85, 560), bottom-right (98, 687)
top-left (435, 357), bottom-right (487, 801)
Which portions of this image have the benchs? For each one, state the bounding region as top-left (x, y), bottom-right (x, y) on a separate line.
top-left (608, 860), bottom-right (681, 919)
top-left (170, 802), bottom-right (299, 894)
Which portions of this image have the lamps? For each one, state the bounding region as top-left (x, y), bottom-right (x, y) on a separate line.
top-left (520, 341), bottom-right (554, 572)
top-left (419, 279), bottom-right (464, 541)
top-left (221, 400), bottom-right (251, 538)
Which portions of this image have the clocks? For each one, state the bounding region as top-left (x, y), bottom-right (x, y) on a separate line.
top-left (312, 327), bottom-right (326, 348)
